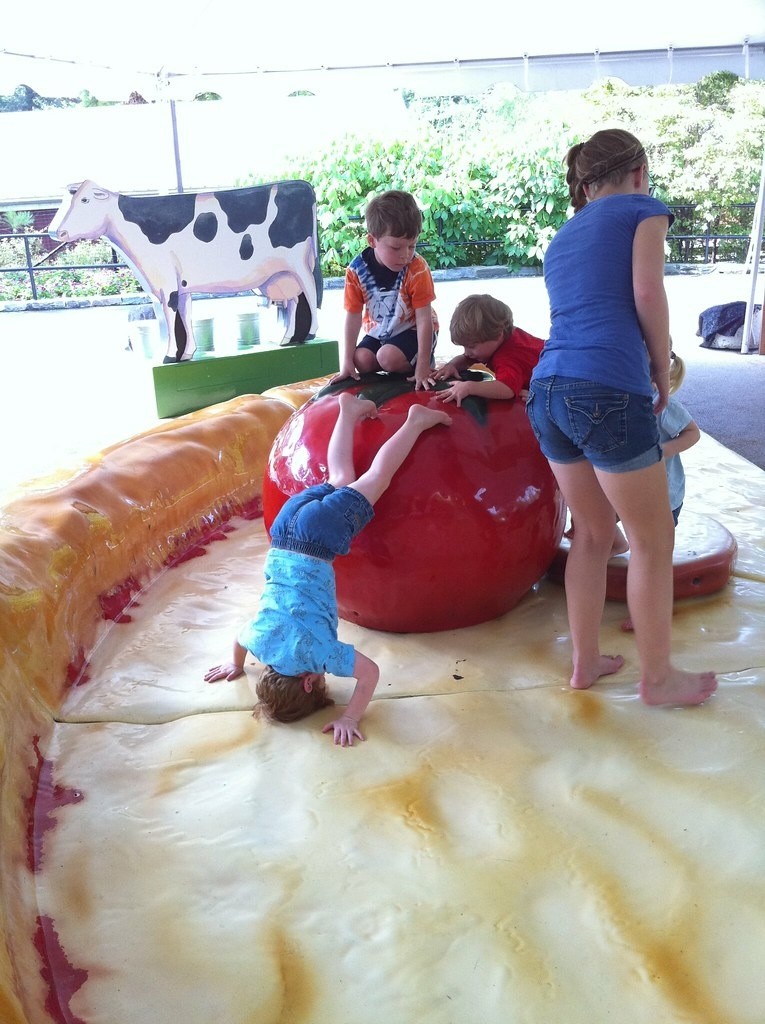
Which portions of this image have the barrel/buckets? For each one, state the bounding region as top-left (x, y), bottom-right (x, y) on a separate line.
top-left (192, 318), bottom-right (213, 352)
top-left (126, 319), bottom-right (160, 357)
top-left (234, 312), bottom-right (261, 345)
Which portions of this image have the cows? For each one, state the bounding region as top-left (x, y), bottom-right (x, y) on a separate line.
top-left (47, 178), bottom-right (324, 365)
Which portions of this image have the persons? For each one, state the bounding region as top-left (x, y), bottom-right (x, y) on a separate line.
top-left (328, 191), bottom-right (440, 390)
top-left (525, 129), bottom-right (718, 705)
top-left (623, 336), bottom-right (701, 630)
top-left (204, 392), bottom-right (453, 745)
top-left (429, 294), bottom-right (628, 558)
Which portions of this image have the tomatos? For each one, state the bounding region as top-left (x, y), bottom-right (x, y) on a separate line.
top-left (261, 367), bottom-right (570, 634)
